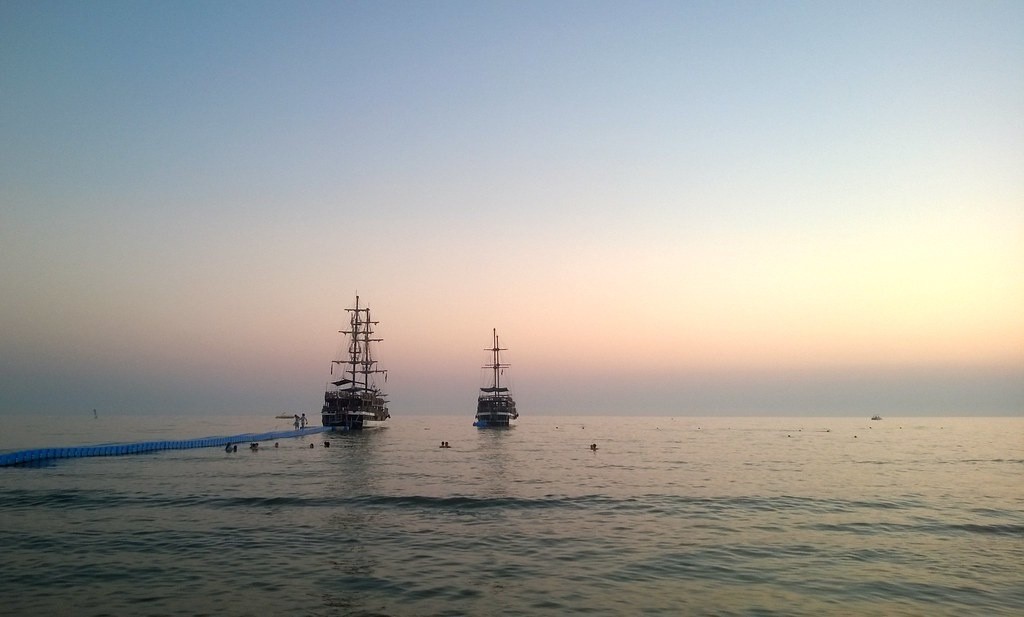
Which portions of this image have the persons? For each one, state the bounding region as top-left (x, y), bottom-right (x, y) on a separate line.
top-left (293, 414), bottom-right (300, 429)
top-left (299, 414), bottom-right (308, 428)
top-left (590, 444), bottom-right (597, 451)
top-left (439, 442), bottom-right (452, 449)
top-left (224, 442), bottom-right (331, 451)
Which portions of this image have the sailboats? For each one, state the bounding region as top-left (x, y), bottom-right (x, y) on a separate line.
top-left (322, 294), bottom-right (390, 428)
top-left (475, 324), bottom-right (518, 429)
